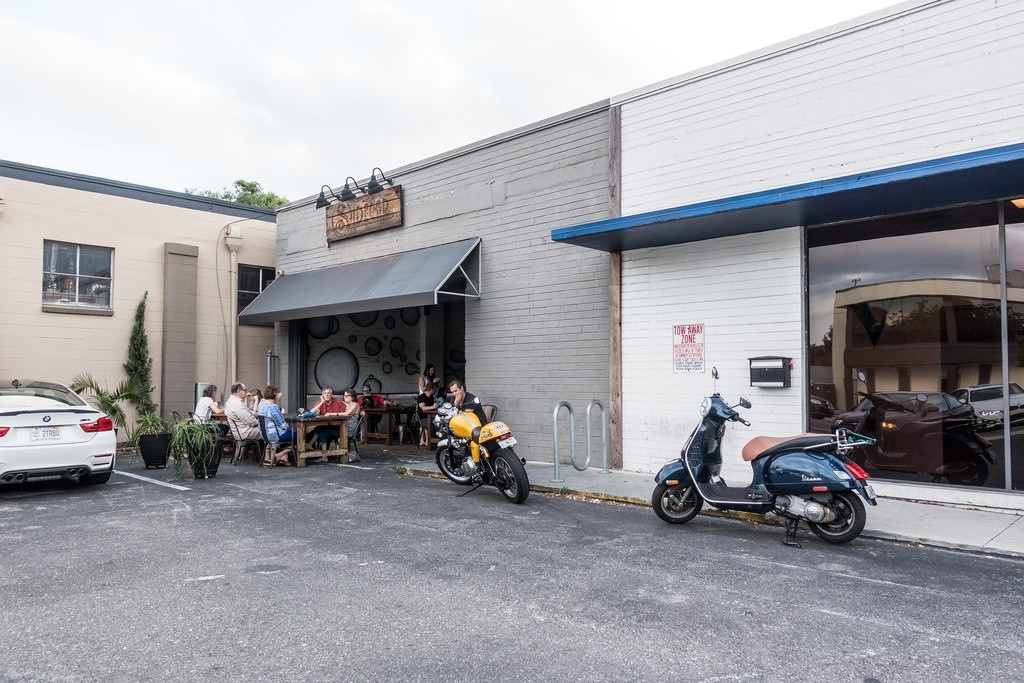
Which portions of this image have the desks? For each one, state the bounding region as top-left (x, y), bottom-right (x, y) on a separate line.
top-left (209, 413), bottom-right (353, 468)
top-left (423, 410), bottom-right (441, 451)
top-left (361, 405), bottom-right (416, 445)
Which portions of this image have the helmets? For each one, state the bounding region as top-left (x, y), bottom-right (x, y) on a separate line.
top-left (362, 396), bottom-right (373, 408)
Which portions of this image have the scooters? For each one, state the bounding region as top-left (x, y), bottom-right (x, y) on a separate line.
top-left (843, 370), bottom-right (997, 486)
top-left (652, 367), bottom-right (877, 547)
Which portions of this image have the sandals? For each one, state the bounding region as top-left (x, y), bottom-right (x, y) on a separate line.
top-left (278, 459), bottom-right (292, 467)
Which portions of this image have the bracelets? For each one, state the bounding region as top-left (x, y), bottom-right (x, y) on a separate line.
top-left (337, 413), bottom-right (338, 416)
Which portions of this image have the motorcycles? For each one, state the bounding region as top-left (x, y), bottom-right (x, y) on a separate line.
top-left (433, 395), bottom-right (530, 504)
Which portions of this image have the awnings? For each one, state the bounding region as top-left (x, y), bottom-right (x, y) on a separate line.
top-left (551, 143), bottom-right (1024, 251)
top-left (238, 235), bottom-right (481, 326)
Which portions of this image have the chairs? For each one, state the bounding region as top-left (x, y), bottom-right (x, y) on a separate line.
top-left (392, 404), bottom-right (417, 444)
top-left (188, 412), bottom-right (233, 459)
top-left (357, 414), bottom-right (383, 443)
top-left (224, 416), bottom-right (264, 466)
top-left (172, 410), bottom-right (183, 423)
top-left (255, 415), bottom-right (297, 470)
top-left (481, 404), bottom-right (498, 424)
top-left (991, 390), bottom-right (1001, 397)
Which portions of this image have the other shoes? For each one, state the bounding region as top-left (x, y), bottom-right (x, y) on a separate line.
top-left (313, 459), bottom-right (327, 464)
top-left (262, 458), bottom-right (276, 465)
top-left (305, 444), bottom-right (314, 451)
top-left (420, 442), bottom-right (426, 446)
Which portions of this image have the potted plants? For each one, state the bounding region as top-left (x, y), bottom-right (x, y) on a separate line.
top-left (129, 410), bottom-right (175, 469)
top-left (169, 413), bottom-right (230, 483)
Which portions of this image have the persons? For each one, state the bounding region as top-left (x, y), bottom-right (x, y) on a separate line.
top-left (309, 386), bottom-right (346, 413)
top-left (305, 388), bottom-right (360, 463)
top-left (417, 382), bottom-right (438, 450)
top-left (450, 380), bottom-right (487, 427)
top-left (225, 383), bottom-right (262, 439)
top-left (357, 384), bottom-right (397, 442)
top-left (194, 385), bottom-right (230, 440)
top-left (418, 363), bottom-right (440, 435)
top-left (437, 387), bottom-right (447, 403)
top-left (248, 388), bottom-right (263, 417)
top-left (257, 386), bottom-right (297, 465)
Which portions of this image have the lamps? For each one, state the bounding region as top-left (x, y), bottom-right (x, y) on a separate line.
top-left (316, 185), bottom-right (341, 210)
top-left (367, 167), bottom-right (394, 195)
top-left (341, 177), bottom-right (366, 202)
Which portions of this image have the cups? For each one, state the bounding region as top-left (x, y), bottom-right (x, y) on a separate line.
top-left (298, 408), bottom-right (305, 417)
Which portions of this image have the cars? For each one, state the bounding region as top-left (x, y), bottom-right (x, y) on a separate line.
top-left (0, 378), bottom-right (117, 490)
top-left (809, 395), bottom-right (833, 419)
top-left (831, 390), bottom-right (968, 441)
top-left (950, 381), bottom-right (1024, 429)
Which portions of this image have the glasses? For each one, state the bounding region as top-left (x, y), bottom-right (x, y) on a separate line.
top-left (239, 389), bottom-right (246, 392)
top-left (344, 395), bottom-right (351, 397)
